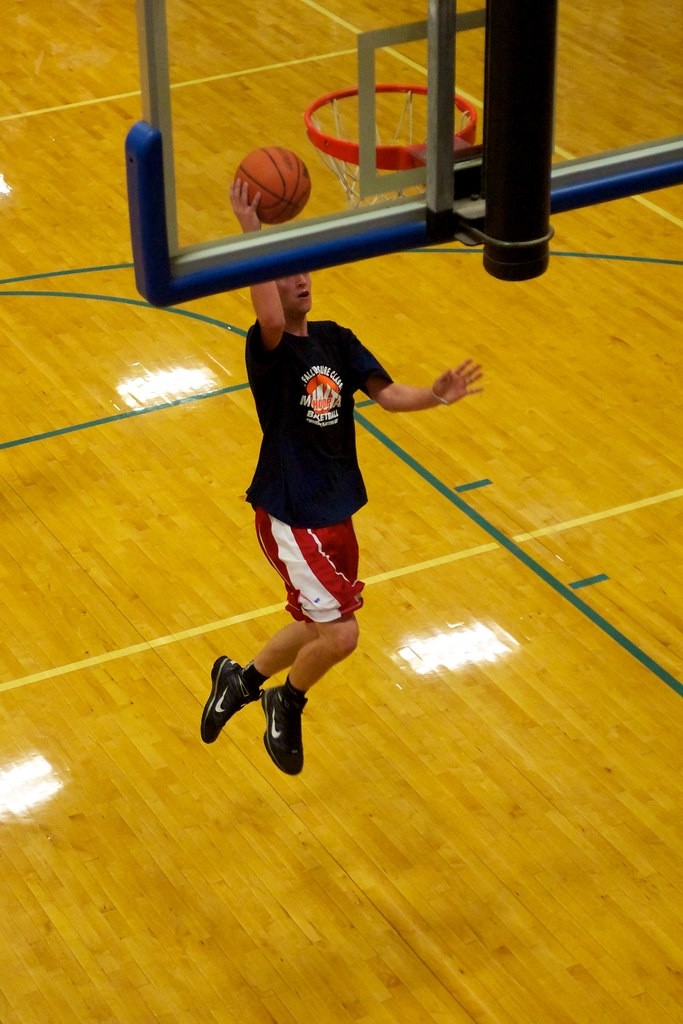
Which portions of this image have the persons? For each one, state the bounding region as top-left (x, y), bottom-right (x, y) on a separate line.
top-left (201, 178), bottom-right (486, 775)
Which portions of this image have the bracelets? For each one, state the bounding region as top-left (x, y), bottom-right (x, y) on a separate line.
top-left (431, 389), bottom-right (450, 405)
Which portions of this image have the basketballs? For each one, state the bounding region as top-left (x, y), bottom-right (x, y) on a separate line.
top-left (231, 147), bottom-right (310, 227)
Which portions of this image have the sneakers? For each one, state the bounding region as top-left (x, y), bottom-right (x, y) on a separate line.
top-left (261, 687), bottom-right (305, 776)
top-left (201, 656), bottom-right (259, 742)
